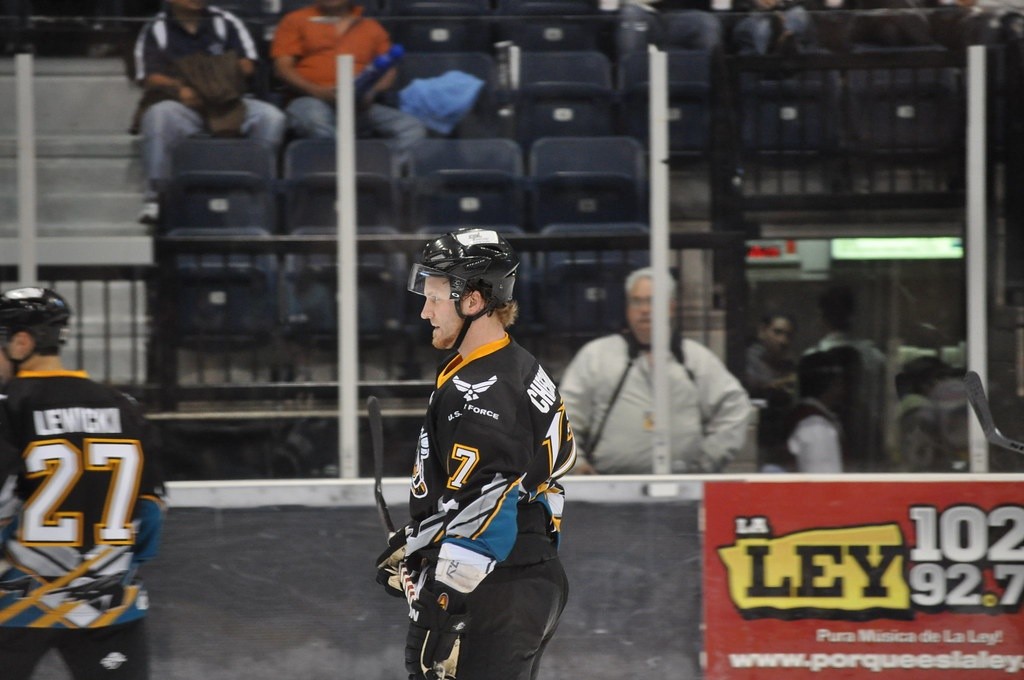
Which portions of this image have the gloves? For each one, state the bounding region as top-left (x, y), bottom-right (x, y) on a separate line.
top-left (406, 580), bottom-right (463, 679)
top-left (375, 528), bottom-right (421, 597)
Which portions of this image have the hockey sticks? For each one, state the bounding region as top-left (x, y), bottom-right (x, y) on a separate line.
top-left (366, 395), bottom-right (448, 680)
top-left (961, 369), bottom-right (1024, 455)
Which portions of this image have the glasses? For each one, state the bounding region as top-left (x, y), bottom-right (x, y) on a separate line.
top-left (630, 296), bottom-right (653, 310)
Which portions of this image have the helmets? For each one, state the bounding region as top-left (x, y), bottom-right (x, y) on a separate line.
top-left (0, 288), bottom-right (71, 336)
top-left (409, 228), bottom-right (519, 306)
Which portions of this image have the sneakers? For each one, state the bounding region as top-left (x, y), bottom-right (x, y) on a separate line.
top-left (138, 201), bottom-right (160, 224)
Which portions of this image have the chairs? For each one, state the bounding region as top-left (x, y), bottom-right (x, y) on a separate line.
top-left (136, 2), bottom-right (1023, 347)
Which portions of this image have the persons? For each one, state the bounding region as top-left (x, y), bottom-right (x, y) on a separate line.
top-left (375, 228), bottom-right (577, 680)
top-left (270, 0), bottom-right (424, 170)
top-left (738, 312), bottom-right (800, 473)
top-left (722, 0), bottom-right (810, 50)
top-left (791, 284), bottom-right (895, 473)
top-left (0, 287), bottom-right (166, 680)
top-left (133, 0), bottom-right (284, 224)
top-left (557, 267), bottom-right (752, 475)
